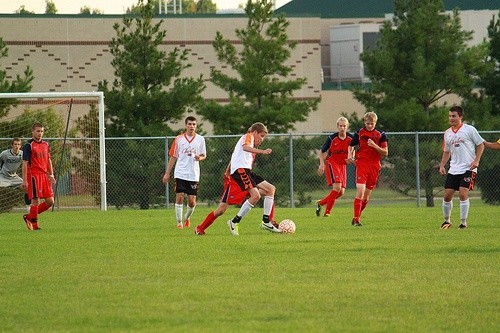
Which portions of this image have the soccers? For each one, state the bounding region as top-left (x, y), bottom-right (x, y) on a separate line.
top-left (278, 219), bottom-right (296, 233)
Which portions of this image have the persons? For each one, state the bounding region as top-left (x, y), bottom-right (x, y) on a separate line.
top-left (194, 126), bottom-right (279, 234)
top-left (22, 123), bottom-right (55, 230)
top-left (228, 122), bottom-right (280, 236)
top-left (314, 117), bottom-right (355, 216)
top-left (162, 116), bottom-right (206, 227)
top-left (439, 106), bottom-right (485, 230)
top-left (484, 138), bottom-right (500, 149)
top-left (0, 138), bottom-right (31, 212)
top-left (348, 112), bottom-right (389, 226)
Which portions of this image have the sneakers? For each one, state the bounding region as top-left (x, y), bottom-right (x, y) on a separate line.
top-left (23, 214), bottom-right (41, 230)
top-left (228, 220), bottom-right (240, 236)
top-left (177, 222), bottom-right (183, 228)
top-left (458, 224), bottom-right (469, 230)
top-left (323, 214), bottom-right (331, 219)
top-left (440, 221), bottom-right (452, 229)
top-left (185, 220), bottom-right (191, 227)
top-left (195, 225), bottom-right (205, 235)
top-left (352, 218), bottom-right (362, 226)
top-left (270, 218), bottom-right (279, 229)
top-left (314, 200), bottom-right (323, 217)
top-left (261, 221), bottom-right (282, 232)
